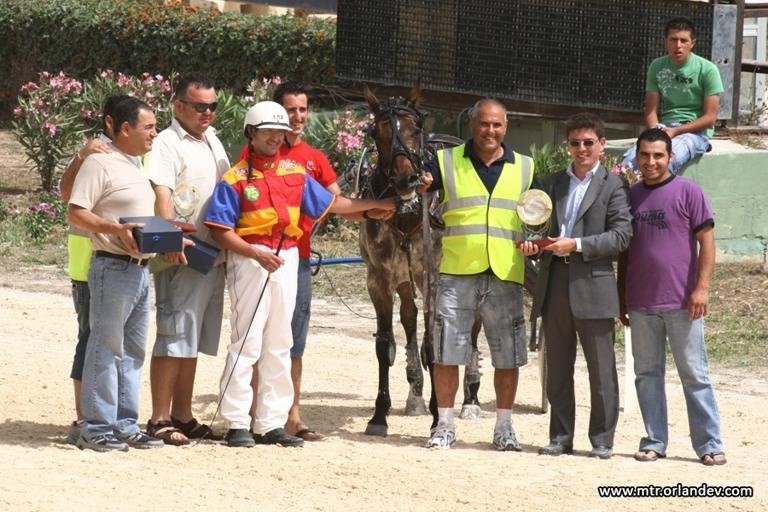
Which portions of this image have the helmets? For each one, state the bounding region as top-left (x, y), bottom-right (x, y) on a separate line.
top-left (243, 101), bottom-right (293, 138)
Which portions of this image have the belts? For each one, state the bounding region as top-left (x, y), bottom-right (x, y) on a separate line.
top-left (552, 255), bottom-right (578, 265)
top-left (95, 249), bottom-right (149, 267)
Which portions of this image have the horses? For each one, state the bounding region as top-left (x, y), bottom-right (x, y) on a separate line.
top-left (360, 76), bottom-right (482, 438)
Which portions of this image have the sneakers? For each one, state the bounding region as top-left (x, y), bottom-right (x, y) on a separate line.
top-left (426, 419), bottom-right (457, 452)
top-left (69, 419), bottom-right (131, 451)
top-left (250, 425), bottom-right (305, 448)
top-left (108, 425), bottom-right (166, 451)
top-left (226, 422), bottom-right (255, 449)
top-left (492, 420), bottom-right (523, 452)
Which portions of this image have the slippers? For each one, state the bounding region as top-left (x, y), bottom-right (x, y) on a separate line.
top-left (633, 438), bottom-right (667, 463)
top-left (700, 440), bottom-right (728, 466)
top-left (282, 421), bottom-right (328, 442)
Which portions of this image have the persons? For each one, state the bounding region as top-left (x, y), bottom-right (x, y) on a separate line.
top-left (619, 16), bottom-right (725, 177)
top-left (519, 112), bottom-right (634, 459)
top-left (416, 98), bottom-right (541, 451)
top-left (617, 128), bottom-right (726, 466)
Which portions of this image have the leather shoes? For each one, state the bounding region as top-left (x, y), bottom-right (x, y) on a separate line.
top-left (538, 439), bottom-right (574, 457)
top-left (588, 440), bottom-right (614, 460)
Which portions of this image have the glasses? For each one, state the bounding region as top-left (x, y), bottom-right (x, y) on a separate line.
top-left (181, 100), bottom-right (218, 113)
top-left (570, 137), bottom-right (600, 146)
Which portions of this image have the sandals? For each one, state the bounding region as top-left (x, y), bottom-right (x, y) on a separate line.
top-left (146, 417), bottom-right (191, 447)
top-left (169, 416), bottom-right (225, 440)
